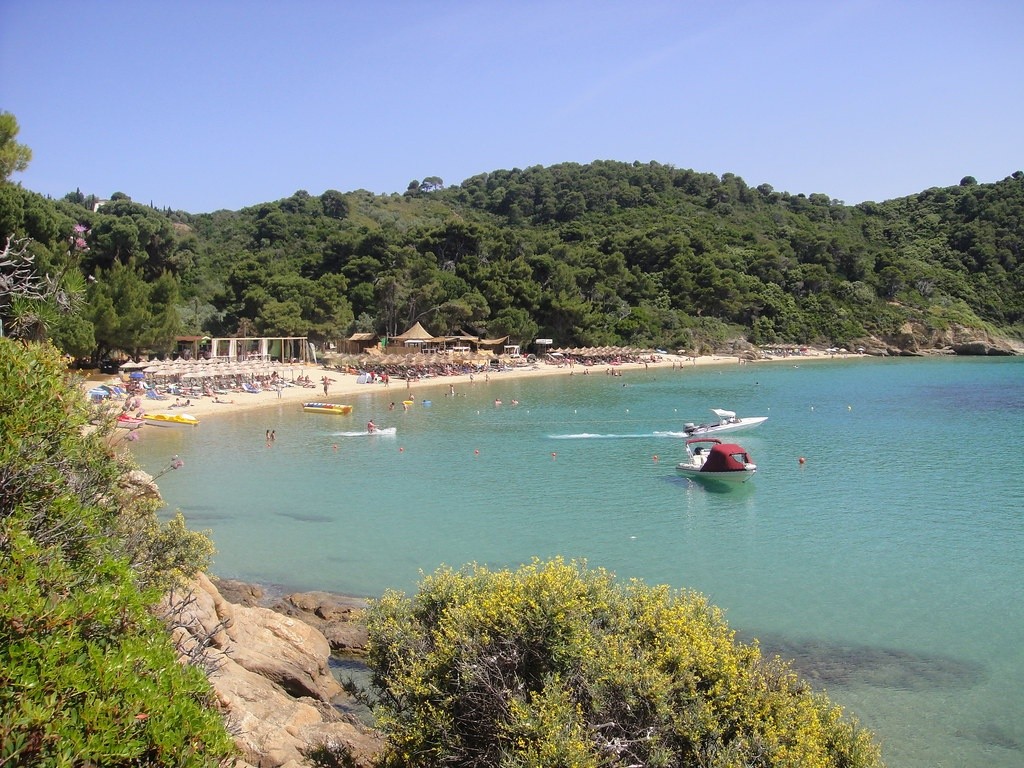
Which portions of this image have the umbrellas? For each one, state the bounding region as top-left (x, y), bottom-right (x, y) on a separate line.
top-left (323, 350), bottom-right (513, 367)
top-left (89, 384), bottom-right (112, 395)
top-left (120, 355), bottom-right (312, 391)
top-left (758, 342), bottom-right (813, 356)
top-left (825, 346), bottom-right (865, 355)
top-left (547, 344), bottom-right (663, 359)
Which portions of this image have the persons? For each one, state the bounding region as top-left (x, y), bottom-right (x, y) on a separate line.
top-left (495, 398), bottom-right (518, 406)
top-left (723, 417), bottom-right (733, 424)
top-left (409, 393), bottom-right (414, 402)
top-left (266, 430), bottom-right (276, 446)
top-left (168, 397), bottom-right (191, 407)
top-left (389, 403), bottom-right (394, 410)
top-left (212, 397), bottom-right (233, 404)
top-left (262, 371), bottom-right (283, 400)
top-left (564, 354), bottom-right (662, 377)
top-left (349, 364), bottom-right (509, 397)
top-left (367, 420), bottom-right (376, 435)
top-left (667, 355), bottom-right (696, 372)
top-left (296, 373), bottom-right (331, 396)
top-left (738, 357), bottom-right (747, 365)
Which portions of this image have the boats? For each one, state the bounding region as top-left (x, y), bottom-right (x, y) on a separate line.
top-left (92, 413), bottom-right (144, 431)
top-left (676, 438), bottom-right (758, 483)
top-left (141, 413), bottom-right (200, 430)
top-left (300, 400), bottom-right (353, 416)
top-left (682, 409), bottom-right (769, 440)
